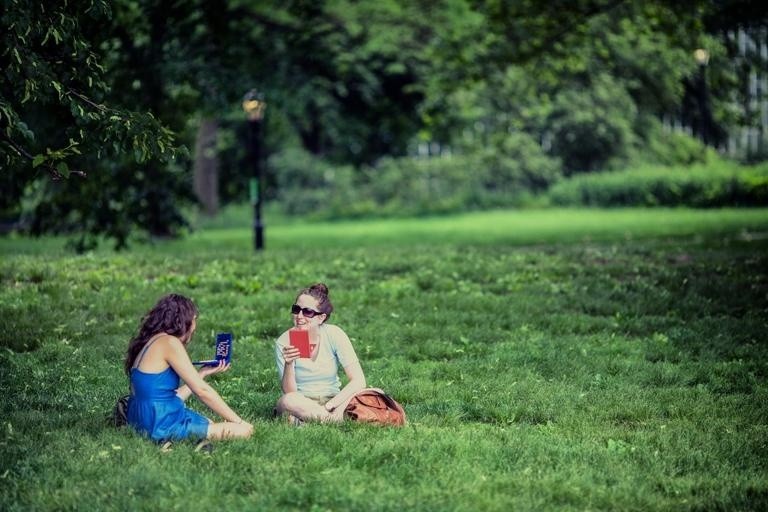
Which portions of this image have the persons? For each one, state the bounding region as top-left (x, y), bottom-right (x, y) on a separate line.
top-left (124, 294), bottom-right (253, 447)
top-left (273, 283), bottom-right (366, 427)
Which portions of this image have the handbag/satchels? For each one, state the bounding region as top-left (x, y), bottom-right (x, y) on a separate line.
top-left (343, 387), bottom-right (408, 426)
top-left (114, 395), bottom-right (132, 427)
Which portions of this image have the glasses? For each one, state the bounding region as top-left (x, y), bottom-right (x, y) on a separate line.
top-left (290, 302), bottom-right (323, 319)
